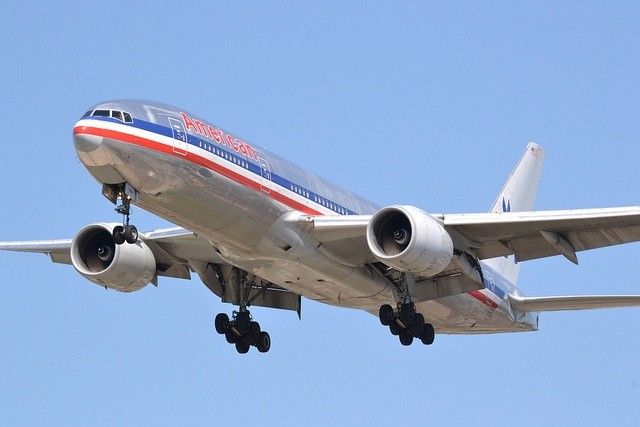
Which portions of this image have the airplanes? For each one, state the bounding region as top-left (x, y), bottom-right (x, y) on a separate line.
top-left (0, 98), bottom-right (640, 355)
